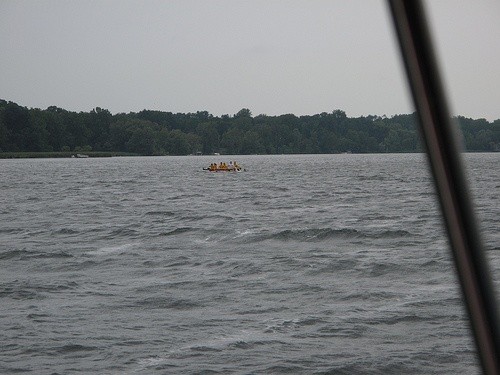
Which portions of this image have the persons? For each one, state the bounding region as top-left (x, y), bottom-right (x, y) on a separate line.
top-left (209, 160), bottom-right (242, 172)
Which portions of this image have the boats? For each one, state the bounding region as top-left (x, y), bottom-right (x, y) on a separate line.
top-left (202, 166), bottom-right (242, 172)
